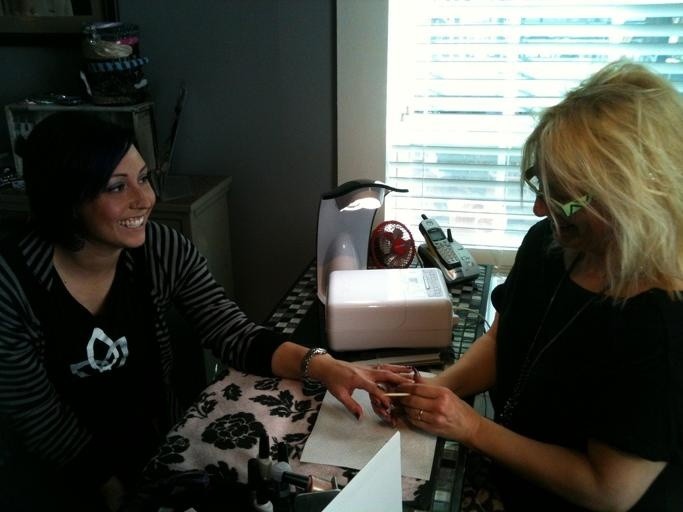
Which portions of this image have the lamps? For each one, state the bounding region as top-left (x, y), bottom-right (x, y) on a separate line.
top-left (315, 179), bottom-right (409, 309)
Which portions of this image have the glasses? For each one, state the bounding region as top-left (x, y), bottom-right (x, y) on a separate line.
top-left (523, 167), bottom-right (592, 222)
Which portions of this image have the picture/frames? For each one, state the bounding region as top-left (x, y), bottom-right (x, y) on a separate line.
top-left (0, 1), bottom-right (120, 48)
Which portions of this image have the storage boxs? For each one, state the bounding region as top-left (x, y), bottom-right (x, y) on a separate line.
top-left (323, 267), bottom-right (456, 352)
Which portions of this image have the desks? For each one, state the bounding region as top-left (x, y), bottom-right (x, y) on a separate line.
top-left (150, 256), bottom-right (492, 512)
top-left (0, 175), bottom-right (235, 309)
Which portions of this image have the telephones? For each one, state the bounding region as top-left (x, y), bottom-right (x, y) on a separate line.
top-left (418, 214), bottom-right (480, 285)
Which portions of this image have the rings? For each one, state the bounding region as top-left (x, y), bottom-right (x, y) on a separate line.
top-left (416, 408), bottom-right (425, 422)
top-left (375, 359), bottom-right (383, 371)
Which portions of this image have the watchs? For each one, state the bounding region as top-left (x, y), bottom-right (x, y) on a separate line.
top-left (300, 347), bottom-right (326, 385)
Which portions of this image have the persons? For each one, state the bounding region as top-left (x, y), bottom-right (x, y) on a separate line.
top-left (367, 55), bottom-right (683, 511)
top-left (1, 110), bottom-right (413, 511)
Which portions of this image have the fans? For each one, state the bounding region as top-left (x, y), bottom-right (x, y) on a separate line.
top-left (368, 217), bottom-right (416, 269)
